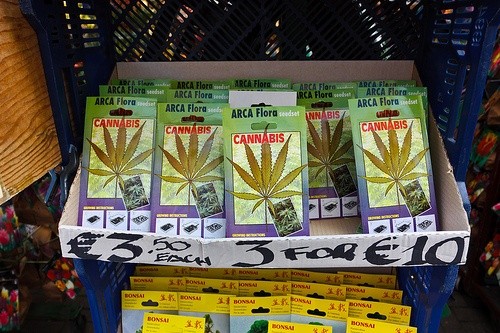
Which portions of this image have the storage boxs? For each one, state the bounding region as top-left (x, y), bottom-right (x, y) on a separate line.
top-left (17, 0), bottom-right (500, 333)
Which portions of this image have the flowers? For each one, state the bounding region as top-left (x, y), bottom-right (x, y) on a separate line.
top-left (465, 45), bottom-right (500, 291)
top-left (0, 169), bottom-right (83, 332)
